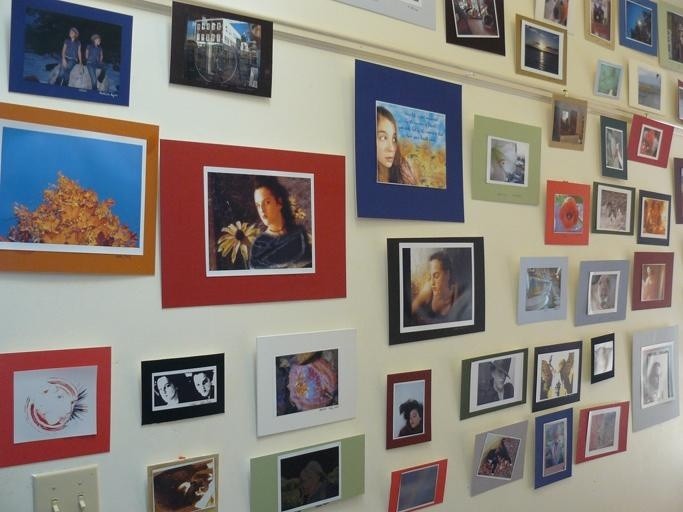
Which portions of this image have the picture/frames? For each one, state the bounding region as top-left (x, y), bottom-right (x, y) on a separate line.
top-left (445, 0), bottom-right (505, 56)
top-left (170, 0), bottom-right (274, 98)
top-left (250, 434), bottom-right (365, 512)
top-left (8, 0), bottom-right (133, 107)
top-left (141, 352), bottom-right (225, 426)
top-left (355, 58), bottom-right (464, 222)
top-left (388, 459), bottom-right (448, 512)
top-left (256, 329), bottom-right (357, 438)
top-left (386, 369), bottom-right (431, 450)
top-left (147, 453), bottom-right (220, 512)
top-left (335, 0), bottom-right (436, 31)
top-left (1, 102), bottom-right (160, 276)
top-left (387, 236), bottom-right (486, 346)
top-left (160, 139), bottom-right (348, 308)
top-left (0, 346), bottom-right (112, 469)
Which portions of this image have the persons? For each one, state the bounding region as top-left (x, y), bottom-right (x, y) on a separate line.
top-left (248, 174), bottom-right (311, 268)
top-left (86, 35), bottom-right (105, 91)
top-left (479, 358), bottom-right (514, 405)
top-left (374, 108), bottom-right (417, 183)
top-left (398, 400), bottom-right (422, 434)
top-left (593, 0), bottom-right (605, 35)
top-left (608, 132), bottom-right (623, 170)
top-left (548, 355), bottom-right (567, 399)
top-left (192, 370), bottom-right (214, 401)
top-left (644, 354), bottom-right (666, 402)
top-left (153, 378), bottom-right (195, 407)
top-left (481, 438), bottom-right (513, 479)
top-left (642, 266), bottom-right (659, 298)
top-left (298, 455), bottom-right (334, 505)
top-left (54, 27), bottom-right (82, 86)
top-left (412, 250), bottom-right (462, 324)
top-left (594, 344), bottom-right (609, 375)
